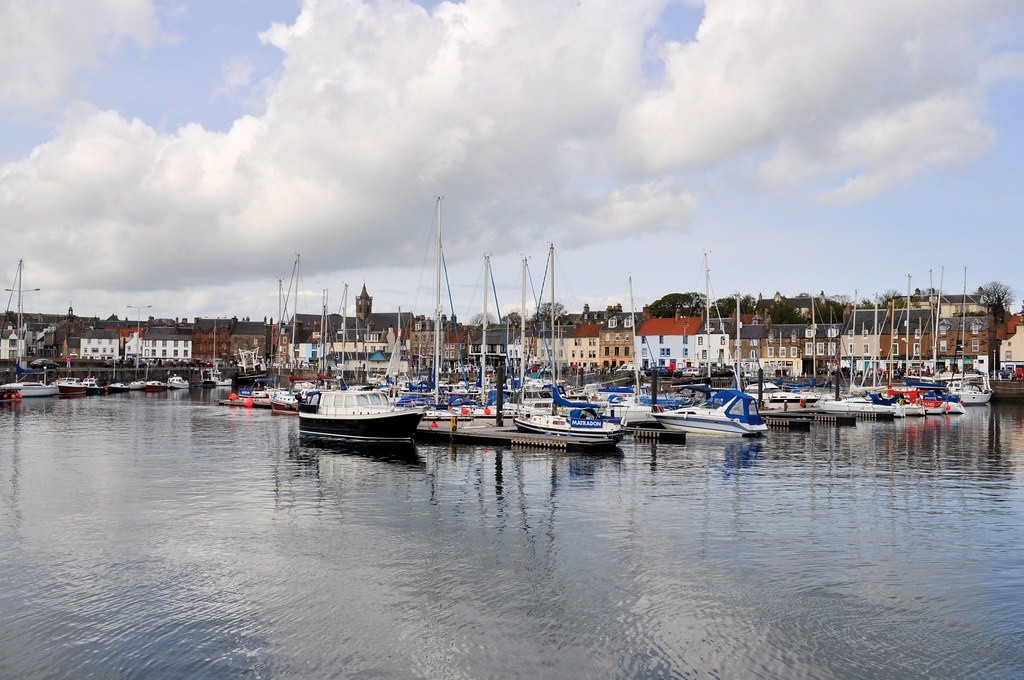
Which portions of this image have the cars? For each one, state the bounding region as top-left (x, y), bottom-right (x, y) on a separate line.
top-left (30, 359), bottom-right (61, 370)
top-left (103, 357), bottom-right (223, 368)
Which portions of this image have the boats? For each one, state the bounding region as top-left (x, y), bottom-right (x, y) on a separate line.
top-left (105, 381), bottom-right (168, 393)
top-left (166, 373), bottom-right (190, 389)
top-left (202, 380), bottom-right (216, 389)
top-left (0, 380), bottom-right (61, 397)
top-left (294, 384), bottom-right (430, 440)
top-left (57, 376), bottom-right (105, 397)
top-left (207, 368), bottom-right (233, 386)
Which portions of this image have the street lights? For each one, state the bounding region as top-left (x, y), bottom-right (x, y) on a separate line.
top-left (126, 305), bottom-right (153, 368)
top-left (4, 288), bottom-right (41, 372)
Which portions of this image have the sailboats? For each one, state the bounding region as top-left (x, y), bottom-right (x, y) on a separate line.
top-left (193, 195), bottom-right (996, 448)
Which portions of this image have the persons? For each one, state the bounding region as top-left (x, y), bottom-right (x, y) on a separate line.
top-left (118, 358), bottom-right (202, 369)
top-left (431, 422), bottom-right (438, 427)
top-left (55, 375), bottom-right (62, 384)
top-left (580, 412), bottom-right (590, 419)
top-left (66, 356), bottom-right (71, 368)
top-left (485, 407), bottom-right (490, 415)
